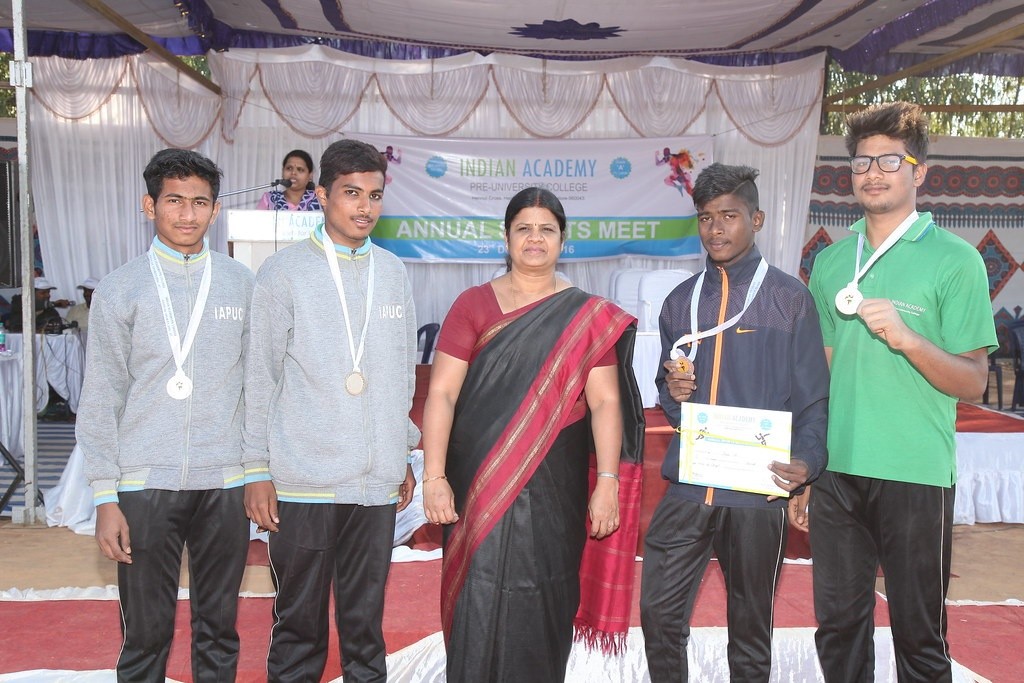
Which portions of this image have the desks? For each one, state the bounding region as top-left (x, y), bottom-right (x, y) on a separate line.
top-left (0, 329), bottom-right (88, 467)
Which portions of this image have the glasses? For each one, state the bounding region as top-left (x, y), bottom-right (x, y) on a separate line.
top-left (848, 154), bottom-right (917, 174)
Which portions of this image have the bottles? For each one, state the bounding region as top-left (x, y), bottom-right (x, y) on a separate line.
top-left (0, 323), bottom-right (6, 352)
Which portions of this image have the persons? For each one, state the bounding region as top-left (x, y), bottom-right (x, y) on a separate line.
top-left (75, 148), bottom-right (256, 683)
top-left (786, 102), bottom-right (1001, 683)
top-left (422, 188), bottom-right (624, 683)
top-left (256, 149), bottom-right (323, 211)
top-left (12, 277), bottom-right (69, 415)
top-left (77, 278), bottom-right (98, 308)
top-left (639, 163), bottom-right (831, 683)
top-left (241, 139), bottom-right (419, 683)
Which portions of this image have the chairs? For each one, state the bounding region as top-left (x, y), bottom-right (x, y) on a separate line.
top-left (1009, 320), bottom-right (1024, 409)
top-left (417, 322), bottom-right (441, 363)
top-left (982, 351), bottom-right (1006, 410)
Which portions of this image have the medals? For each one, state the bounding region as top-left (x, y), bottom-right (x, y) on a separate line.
top-left (671, 356), bottom-right (694, 375)
top-left (835, 287), bottom-right (864, 315)
top-left (344, 372), bottom-right (367, 398)
top-left (167, 376), bottom-right (193, 399)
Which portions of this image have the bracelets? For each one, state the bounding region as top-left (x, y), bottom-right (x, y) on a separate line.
top-left (423, 476), bottom-right (448, 483)
top-left (597, 472), bottom-right (618, 480)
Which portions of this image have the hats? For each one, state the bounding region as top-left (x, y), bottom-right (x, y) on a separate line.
top-left (34, 277), bottom-right (57, 290)
top-left (76, 277), bottom-right (99, 290)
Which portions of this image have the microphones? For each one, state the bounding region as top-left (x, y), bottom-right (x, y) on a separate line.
top-left (276, 179), bottom-right (292, 187)
